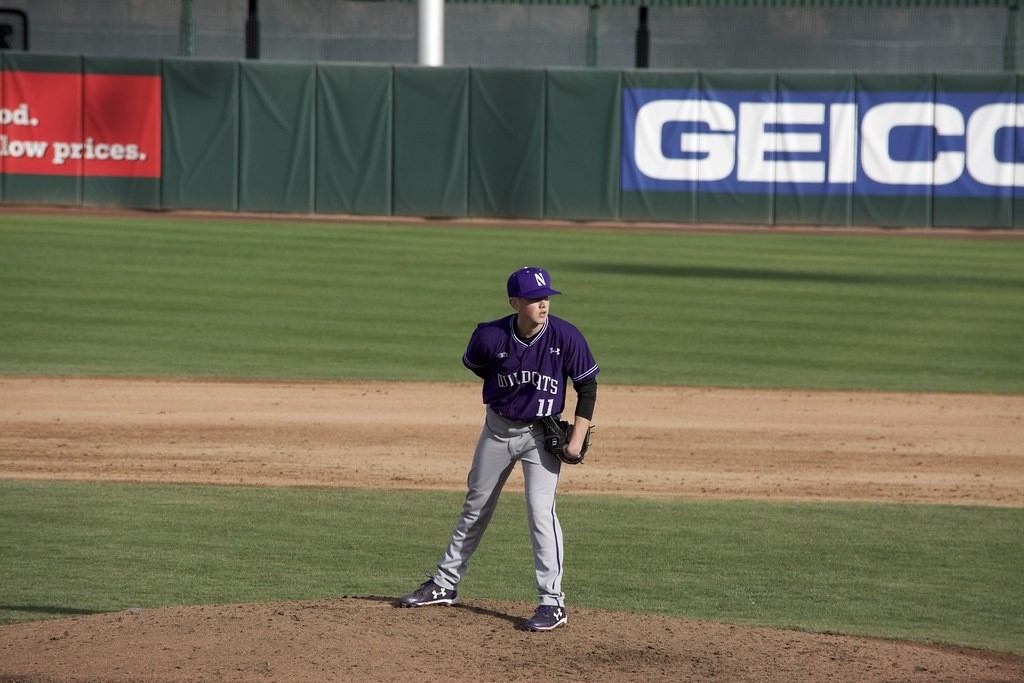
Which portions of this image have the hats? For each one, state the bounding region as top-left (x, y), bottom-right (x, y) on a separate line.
top-left (507, 267), bottom-right (561, 299)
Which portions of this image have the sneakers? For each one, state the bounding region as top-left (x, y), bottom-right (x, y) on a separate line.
top-left (400, 570), bottom-right (458, 608)
top-left (526, 605), bottom-right (567, 632)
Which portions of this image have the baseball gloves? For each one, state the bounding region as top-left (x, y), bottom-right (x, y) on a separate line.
top-left (542, 415), bottom-right (591, 465)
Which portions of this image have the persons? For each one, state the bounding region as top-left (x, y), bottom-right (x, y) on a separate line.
top-left (402, 266), bottom-right (600, 632)
